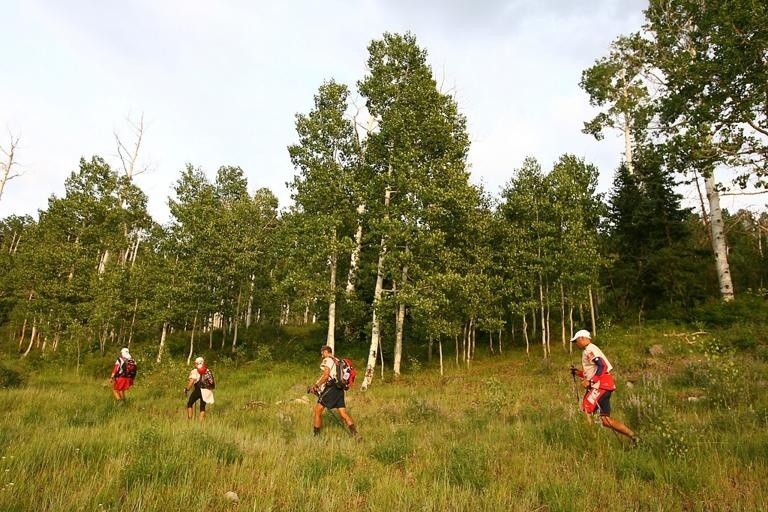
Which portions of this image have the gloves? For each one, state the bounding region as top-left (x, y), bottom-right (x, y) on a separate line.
top-left (183, 388), bottom-right (189, 393)
top-left (570, 365), bottom-right (578, 375)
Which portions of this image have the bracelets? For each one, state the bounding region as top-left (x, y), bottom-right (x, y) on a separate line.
top-left (589, 379), bottom-right (595, 384)
top-left (186, 388), bottom-right (189, 391)
top-left (314, 384), bottom-right (320, 390)
top-left (110, 376), bottom-right (114, 380)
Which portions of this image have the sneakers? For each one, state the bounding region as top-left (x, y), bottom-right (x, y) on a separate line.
top-left (624, 435), bottom-right (642, 452)
top-left (117, 399), bottom-right (122, 404)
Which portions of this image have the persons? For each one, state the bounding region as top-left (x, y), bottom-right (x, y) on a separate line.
top-left (307, 345), bottom-right (362, 443)
top-left (109, 348), bottom-right (137, 406)
top-left (183, 357), bottom-right (214, 420)
top-left (570, 329), bottom-right (637, 452)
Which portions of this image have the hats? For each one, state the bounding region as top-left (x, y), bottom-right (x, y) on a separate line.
top-left (196, 357), bottom-right (204, 364)
top-left (121, 348), bottom-right (129, 352)
top-left (570, 330), bottom-right (592, 342)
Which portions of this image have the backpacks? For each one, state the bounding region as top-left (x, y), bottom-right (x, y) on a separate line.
top-left (322, 355), bottom-right (356, 390)
top-left (194, 368), bottom-right (214, 389)
top-left (117, 358), bottom-right (137, 377)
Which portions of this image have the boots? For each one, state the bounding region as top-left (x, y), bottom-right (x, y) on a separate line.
top-left (314, 426), bottom-right (320, 436)
top-left (349, 424), bottom-right (358, 436)
